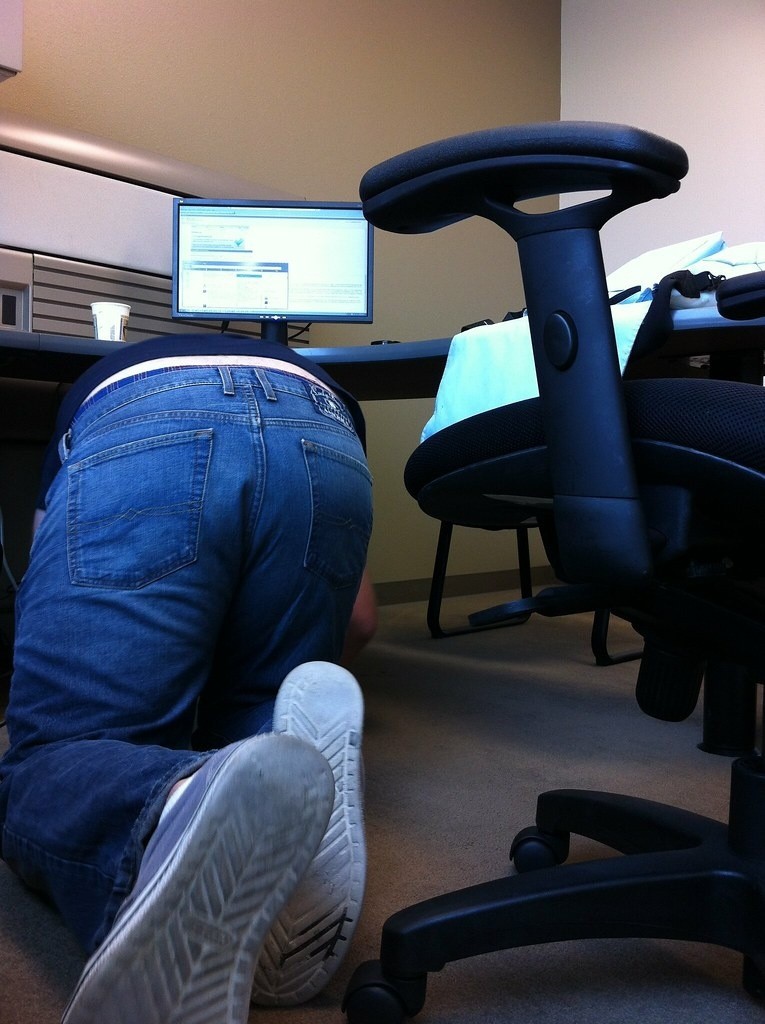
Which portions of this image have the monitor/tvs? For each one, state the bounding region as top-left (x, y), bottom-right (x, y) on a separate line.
top-left (171, 196), bottom-right (373, 345)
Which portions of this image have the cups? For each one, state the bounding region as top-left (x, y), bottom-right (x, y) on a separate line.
top-left (91, 302), bottom-right (131, 342)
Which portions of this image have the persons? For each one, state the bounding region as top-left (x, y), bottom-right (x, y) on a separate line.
top-left (3, 328), bottom-right (378, 1023)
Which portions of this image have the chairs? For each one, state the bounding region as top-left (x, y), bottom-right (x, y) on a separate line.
top-left (341, 120), bottom-right (765, 1023)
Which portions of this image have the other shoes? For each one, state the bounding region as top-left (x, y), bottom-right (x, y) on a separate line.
top-left (60, 733), bottom-right (335, 1024)
top-left (237, 662), bottom-right (367, 1010)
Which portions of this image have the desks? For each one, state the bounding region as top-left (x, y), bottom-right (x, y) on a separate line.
top-left (1, 310), bottom-right (765, 641)
